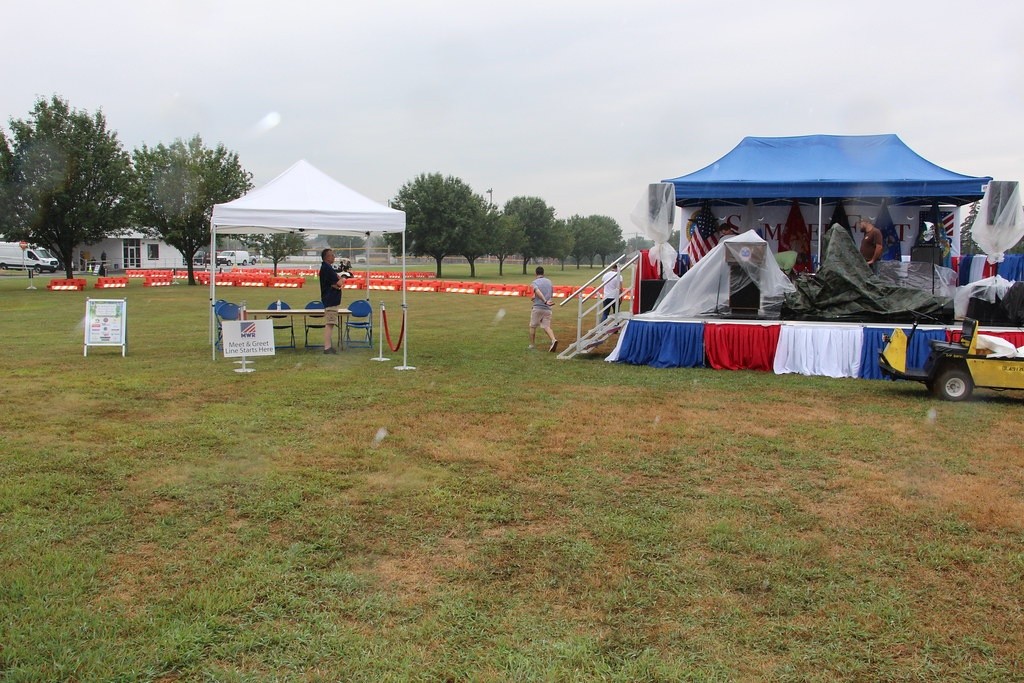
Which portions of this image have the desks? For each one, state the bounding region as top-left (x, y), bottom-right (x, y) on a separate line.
top-left (247, 310), bottom-right (353, 351)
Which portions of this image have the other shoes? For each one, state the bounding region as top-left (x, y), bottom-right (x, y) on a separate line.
top-left (323, 347), bottom-right (337, 354)
top-left (549, 339), bottom-right (559, 353)
top-left (529, 344), bottom-right (533, 348)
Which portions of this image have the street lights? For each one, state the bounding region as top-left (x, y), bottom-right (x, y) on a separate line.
top-left (486, 189), bottom-right (492, 206)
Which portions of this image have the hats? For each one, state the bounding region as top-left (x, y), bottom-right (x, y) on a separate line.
top-left (859, 216), bottom-right (871, 223)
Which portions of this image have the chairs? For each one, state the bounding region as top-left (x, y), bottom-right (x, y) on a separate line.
top-left (215, 299), bottom-right (374, 352)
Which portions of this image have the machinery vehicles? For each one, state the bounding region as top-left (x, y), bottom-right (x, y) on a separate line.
top-left (876, 308), bottom-right (1024, 399)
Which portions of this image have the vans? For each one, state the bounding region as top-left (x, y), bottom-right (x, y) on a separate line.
top-left (0, 242), bottom-right (60, 274)
top-left (217, 251), bottom-right (249, 266)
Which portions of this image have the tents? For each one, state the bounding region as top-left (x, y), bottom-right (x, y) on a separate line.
top-left (660, 134), bottom-right (993, 280)
top-left (209, 160), bottom-right (407, 369)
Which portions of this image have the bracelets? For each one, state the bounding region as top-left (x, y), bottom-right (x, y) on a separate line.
top-left (544, 301), bottom-right (547, 304)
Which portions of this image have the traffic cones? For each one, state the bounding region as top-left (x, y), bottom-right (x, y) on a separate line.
top-left (87, 264), bottom-right (92, 273)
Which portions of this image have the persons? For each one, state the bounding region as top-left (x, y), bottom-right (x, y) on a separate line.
top-left (528, 266), bottom-right (558, 352)
top-left (719, 223), bottom-right (739, 238)
top-left (858, 216), bottom-right (882, 272)
top-left (337, 261), bottom-right (345, 271)
top-left (320, 248), bottom-right (346, 354)
top-left (602, 264), bottom-right (623, 334)
top-left (774, 251), bottom-right (809, 278)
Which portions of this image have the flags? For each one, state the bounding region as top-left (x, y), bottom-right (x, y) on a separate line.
top-left (875, 203), bottom-right (902, 262)
top-left (688, 200), bottom-right (719, 266)
top-left (778, 202), bottom-right (812, 273)
top-left (917, 204), bottom-right (952, 269)
top-left (827, 204), bottom-right (855, 245)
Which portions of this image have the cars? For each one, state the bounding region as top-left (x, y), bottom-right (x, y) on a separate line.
top-left (183, 251), bottom-right (204, 266)
top-left (249, 252), bottom-right (257, 265)
top-left (204, 252), bottom-right (219, 264)
